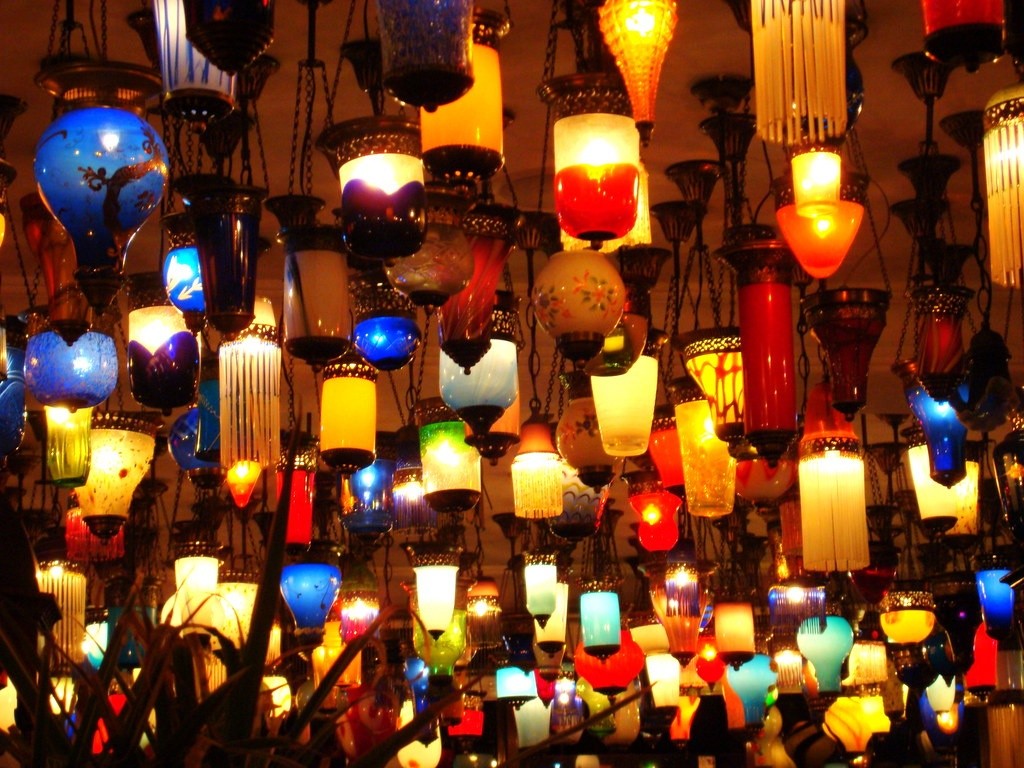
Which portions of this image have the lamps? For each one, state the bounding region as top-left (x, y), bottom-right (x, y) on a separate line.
top-left (0, 0), bottom-right (1024, 768)
